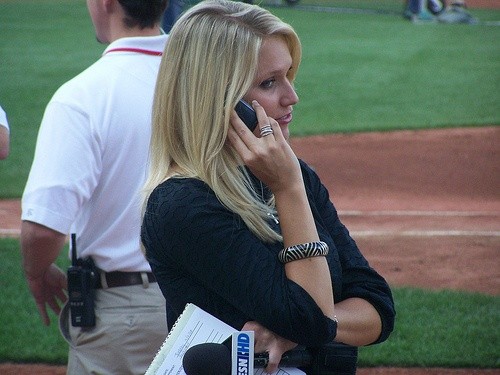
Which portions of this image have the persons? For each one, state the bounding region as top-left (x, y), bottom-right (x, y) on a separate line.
top-left (140, 0), bottom-right (394, 373)
top-left (0, 107), bottom-right (11, 160)
top-left (21, 0), bottom-right (183, 375)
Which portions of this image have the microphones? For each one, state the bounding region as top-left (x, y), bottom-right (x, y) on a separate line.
top-left (182, 331), bottom-right (358, 375)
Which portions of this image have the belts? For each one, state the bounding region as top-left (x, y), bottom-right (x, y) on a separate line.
top-left (93, 271), bottom-right (157, 290)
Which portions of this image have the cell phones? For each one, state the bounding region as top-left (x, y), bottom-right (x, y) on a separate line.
top-left (233, 98), bottom-right (259, 133)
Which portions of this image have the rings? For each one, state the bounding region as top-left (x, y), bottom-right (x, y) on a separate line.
top-left (259, 125), bottom-right (273, 137)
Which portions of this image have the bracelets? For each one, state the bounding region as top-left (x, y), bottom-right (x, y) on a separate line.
top-left (279, 242), bottom-right (331, 266)
